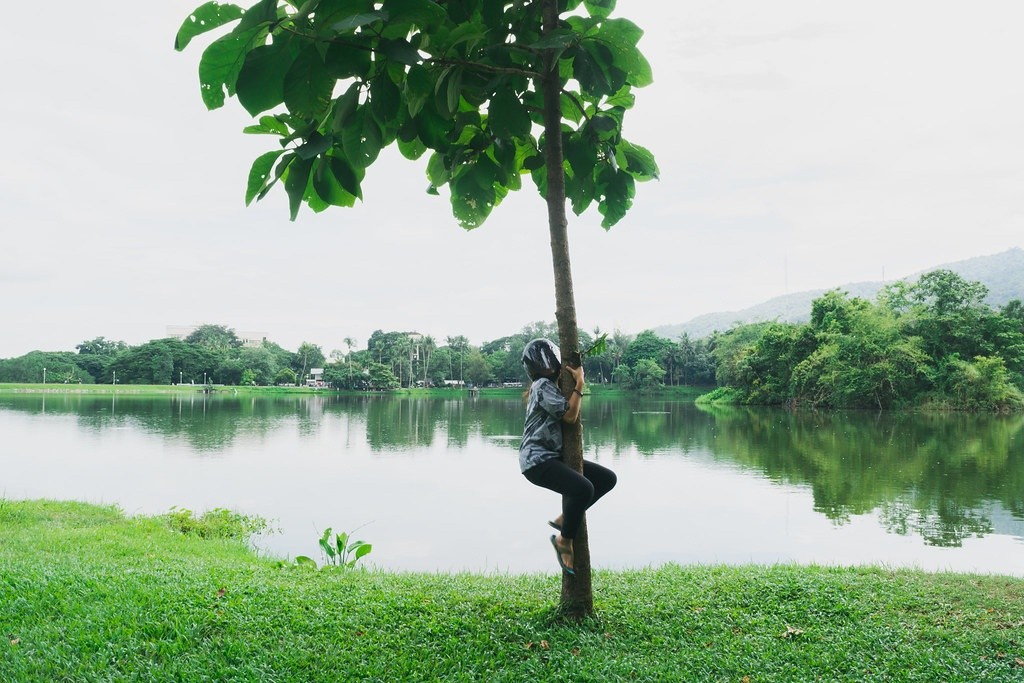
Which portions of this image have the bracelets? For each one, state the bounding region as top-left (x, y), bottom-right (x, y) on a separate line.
top-left (572, 388), bottom-right (583, 398)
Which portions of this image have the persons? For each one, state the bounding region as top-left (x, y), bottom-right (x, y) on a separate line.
top-left (519, 338), bottom-right (617, 576)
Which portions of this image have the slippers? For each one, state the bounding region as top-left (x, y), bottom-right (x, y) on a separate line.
top-left (548, 520), bottom-right (563, 532)
top-left (551, 535), bottom-right (574, 576)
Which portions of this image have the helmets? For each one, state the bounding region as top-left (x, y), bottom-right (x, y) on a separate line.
top-left (520, 338), bottom-right (561, 381)
